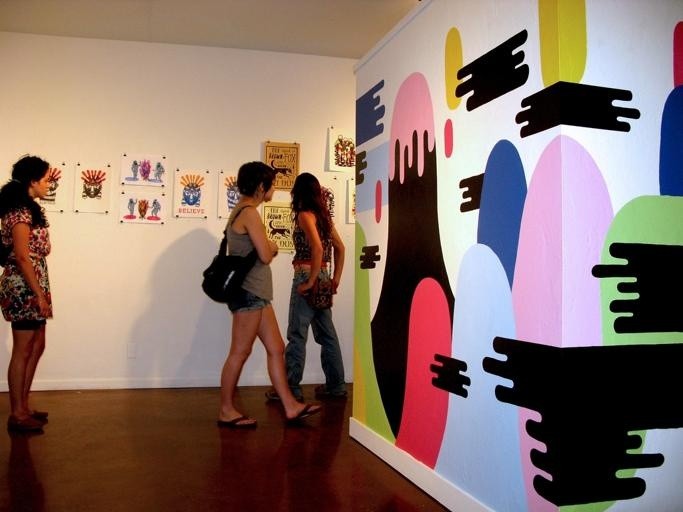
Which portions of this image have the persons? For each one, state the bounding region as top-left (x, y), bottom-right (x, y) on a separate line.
top-left (262, 170), bottom-right (348, 405)
top-left (0, 155), bottom-right (53, 436)
top-left (213, 160), bottom-right (323, 428)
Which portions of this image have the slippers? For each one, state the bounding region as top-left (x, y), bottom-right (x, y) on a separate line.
top-left (285, 402), bottom-right (323, 423)
top-left (216, 414), bottom-right (257, 429)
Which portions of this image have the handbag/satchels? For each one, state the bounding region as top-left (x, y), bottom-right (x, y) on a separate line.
top-left (201, 228), bottom-right (258, 303)
top-left (306, 279), bottom-right (335, 311)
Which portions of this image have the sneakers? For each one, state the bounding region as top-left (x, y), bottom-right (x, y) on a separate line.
top-left (314, 384), bottom-right (348, 399)
top-left (264, 386), bottom-right (304, 401)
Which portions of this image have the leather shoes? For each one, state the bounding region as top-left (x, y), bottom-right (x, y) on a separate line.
top-left (7, 410), bottom-right (50, 432)
